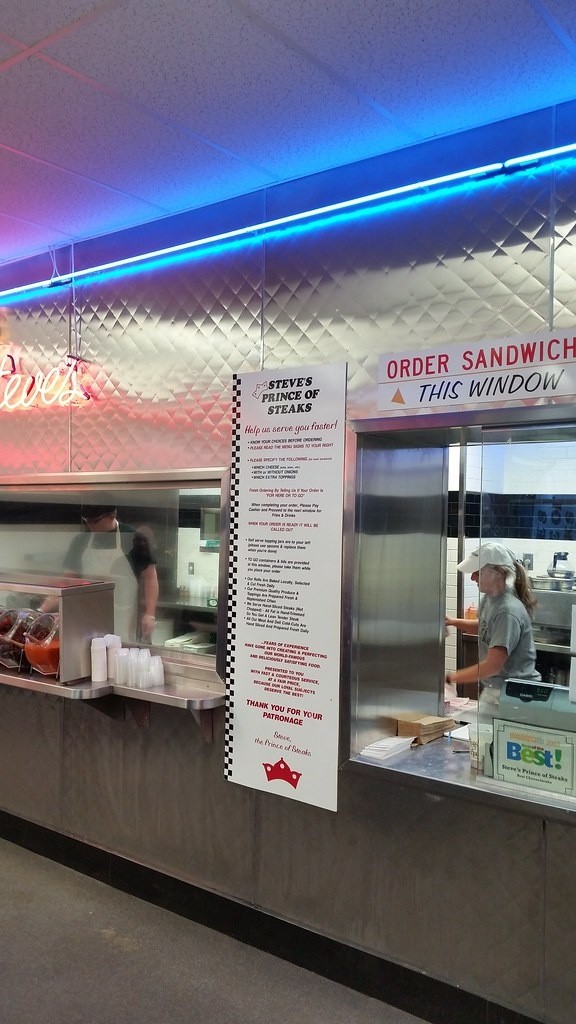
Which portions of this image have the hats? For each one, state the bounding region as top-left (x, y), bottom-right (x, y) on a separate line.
top-left (455, 542), bottom-right (517, 575)
top-left (80, 505), bottom-right (116, 519)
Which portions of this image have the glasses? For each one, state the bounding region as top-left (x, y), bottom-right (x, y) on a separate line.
top-left (80, 512), bottom-right (116, 526)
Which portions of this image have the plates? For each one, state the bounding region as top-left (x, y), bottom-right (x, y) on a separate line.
top-left (165, 631), bottom-right (216, 654)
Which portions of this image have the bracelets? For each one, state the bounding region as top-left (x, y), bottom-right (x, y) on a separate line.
top-left (144, 615), bottom-right (155, 620)
top-left (35, 608), bottom-right (45, 614)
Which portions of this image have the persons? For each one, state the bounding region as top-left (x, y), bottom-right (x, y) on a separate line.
top-left (445, 540), bottom-right (542, 706)
top-left (35, 493), bottom-right (160, 645)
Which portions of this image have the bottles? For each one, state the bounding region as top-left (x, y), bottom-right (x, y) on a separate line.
top-left (178, 585), bottom-right (190, 602)
top-left (467, 602), bottom-right (478, 619)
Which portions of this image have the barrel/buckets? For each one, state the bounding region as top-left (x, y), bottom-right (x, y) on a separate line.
top-left (151, 618), bottom-right (175, 646)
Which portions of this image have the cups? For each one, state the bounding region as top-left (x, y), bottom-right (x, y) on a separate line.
top-left (90, 635), bottom-right (165, 689)
